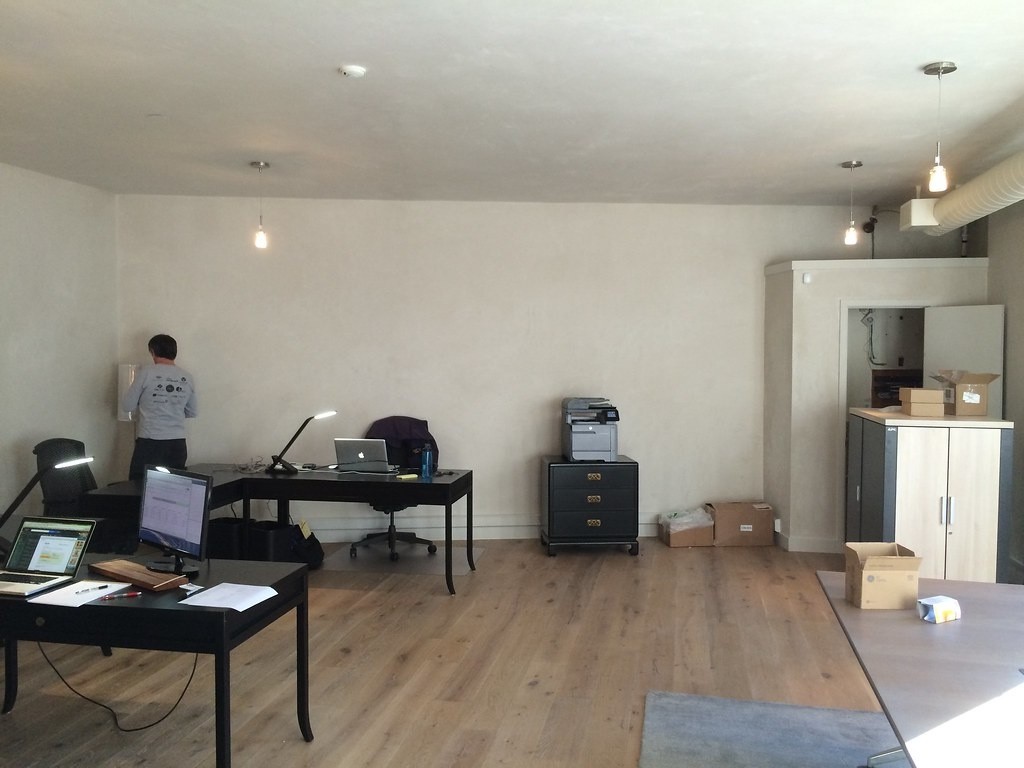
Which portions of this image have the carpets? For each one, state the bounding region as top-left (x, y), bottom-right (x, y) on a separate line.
top-left (639, 692), bottom-right (911, 768)
top-left (322, 544), bottom-right (485, 577)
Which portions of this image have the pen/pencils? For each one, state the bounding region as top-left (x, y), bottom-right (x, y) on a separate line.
top-left (100, 592), bottom-right (142, 600)
top-left (76, 585), bottom-right (108, 593)
top-left (178, 589), bottom-right (198, 601)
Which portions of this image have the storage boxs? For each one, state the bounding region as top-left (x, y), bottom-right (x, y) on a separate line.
top-left (844, 542), bottom-right (923, 610)
top-left (930, 369), bottom-right (1000, 416)
top-left (658, 502), bottom-right (774, 547)
top-left (899, 388), bottom-right (945, 416)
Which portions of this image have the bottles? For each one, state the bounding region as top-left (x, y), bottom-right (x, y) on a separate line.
top-left (422, 443), bottom-right (433, 478)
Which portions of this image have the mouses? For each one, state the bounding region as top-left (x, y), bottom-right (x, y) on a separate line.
top-left (329, 464), bottom-right (338, 469)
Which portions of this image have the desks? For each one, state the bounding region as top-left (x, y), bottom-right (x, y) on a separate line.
top-left (85, 463), bottom-right (475, 594)
top-left (815, 569), bottom-right (1023, 768)
top-left (0, 554), bottom-right (314, 768)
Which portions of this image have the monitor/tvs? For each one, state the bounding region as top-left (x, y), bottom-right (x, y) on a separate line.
top-left (137, 464), bottom-right (214, 576)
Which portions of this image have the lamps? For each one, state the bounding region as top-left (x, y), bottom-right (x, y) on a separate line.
top-left (0, 456), bottom-right (95, 561)
top-left (250, 161), bottom-right (270, 249)
top-left (923, 62), bottom-right (957, 192)
top-left (841, 161), bottom-right (863, 246)
top-left (265, 410), bottom-right (338, 474)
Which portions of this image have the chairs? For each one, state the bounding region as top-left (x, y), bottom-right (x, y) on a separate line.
top-left (349, 416), bottom-right (439, 561)
top-left (33, 438), bottom-right (137, 554)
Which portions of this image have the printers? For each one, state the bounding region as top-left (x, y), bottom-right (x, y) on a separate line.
top-left (560, 397), bottom-right (620, 462)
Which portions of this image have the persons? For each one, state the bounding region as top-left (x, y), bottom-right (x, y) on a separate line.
top-left (121, 334), bottom-right (198, 481)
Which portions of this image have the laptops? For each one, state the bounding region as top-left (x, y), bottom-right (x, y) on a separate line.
top-left (0, 516), bottom-right (96, 596)
top-left (334, 437), bottom-right (399, 472)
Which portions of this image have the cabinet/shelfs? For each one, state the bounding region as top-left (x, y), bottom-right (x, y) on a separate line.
top-left (847, 407), bottom-right (1015, 583)
top-left (539, 454), bottom-right (640, 556)
